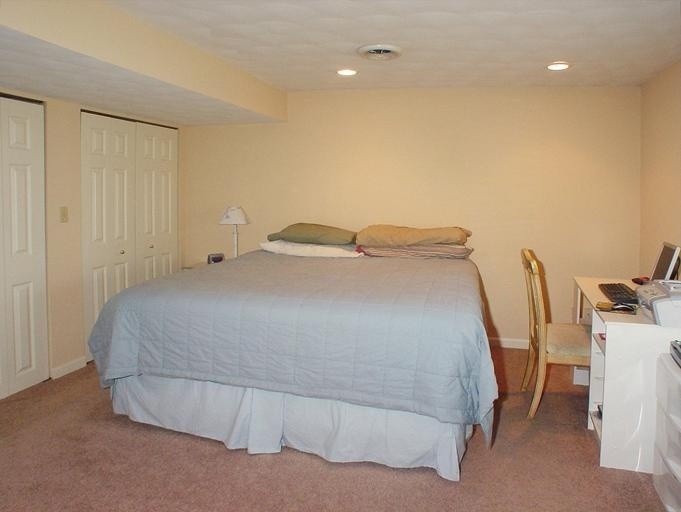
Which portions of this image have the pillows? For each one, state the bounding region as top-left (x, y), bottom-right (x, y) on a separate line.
top-left (360, 245), bottom-right (474, 259)
top-left (354, 224), bottom-right (472, 249)
top-left (258, 238), bottom-right (365, 258)
top-left (267, 223), bottom-right (356, 246)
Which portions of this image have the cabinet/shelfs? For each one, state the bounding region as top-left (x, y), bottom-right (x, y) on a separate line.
top-left (651, 353), bottom-right (681, 511)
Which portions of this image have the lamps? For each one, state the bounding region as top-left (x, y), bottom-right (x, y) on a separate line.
top-left (217, 205), bottom-right (249, 258)
top-left (356, 43), bottom-right (402, 63)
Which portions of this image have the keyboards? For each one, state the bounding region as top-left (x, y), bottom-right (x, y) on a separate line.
top-left (599, 282), bottom-right (639, 304)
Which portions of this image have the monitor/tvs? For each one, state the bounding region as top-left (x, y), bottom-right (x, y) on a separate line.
top-left (649, 240), bottom-right (680, 281)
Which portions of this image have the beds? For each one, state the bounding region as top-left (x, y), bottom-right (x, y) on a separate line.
top-left (87, 250), bottom-right (499, 483)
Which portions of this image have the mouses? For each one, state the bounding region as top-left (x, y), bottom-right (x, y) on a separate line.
top-left (611, 304), bottom-right (635, 313)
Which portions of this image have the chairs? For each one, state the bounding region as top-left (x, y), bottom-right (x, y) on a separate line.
top-left (520, 246), bottom-right (592, 419)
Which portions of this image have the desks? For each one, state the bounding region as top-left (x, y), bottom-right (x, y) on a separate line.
top-left (572, 277), bottom-right (680, 473)
